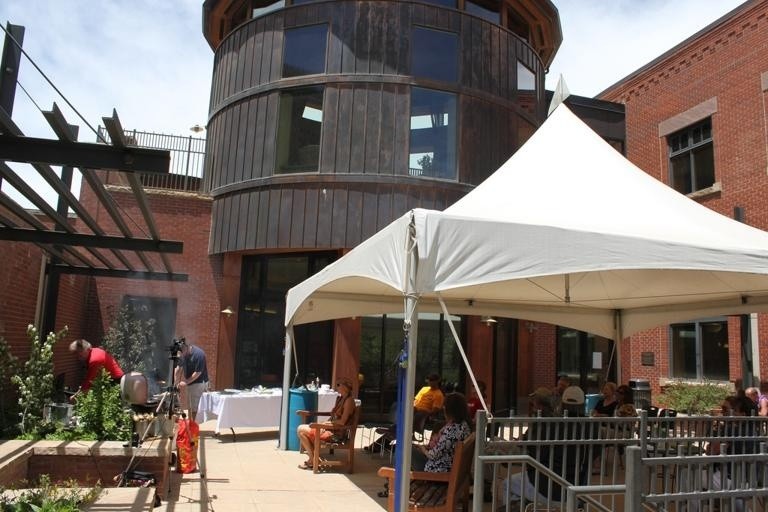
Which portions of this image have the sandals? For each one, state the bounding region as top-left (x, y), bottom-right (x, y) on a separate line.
top-left (298, 461), bottom-right (312, 469)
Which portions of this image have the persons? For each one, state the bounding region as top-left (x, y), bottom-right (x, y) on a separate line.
top-left (66, 336), bottom-right (124, 403)
top-left (172, 343), bottom-right (209, 423)
top-left (296, 376), bottom-right (356, 470)
top-left (363, 372), bottom-right (768, 512)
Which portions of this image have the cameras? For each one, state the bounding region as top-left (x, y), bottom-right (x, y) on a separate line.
top-left (165, 337), bottom-right (184, 356)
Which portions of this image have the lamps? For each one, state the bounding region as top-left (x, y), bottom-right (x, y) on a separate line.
top-left (480, 316), bottom-right (538, 333)
top-left (220, 305), bottom-right (236, 320)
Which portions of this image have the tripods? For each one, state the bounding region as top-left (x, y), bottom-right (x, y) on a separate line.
top-left (116, 386), bottom-right (215, 512)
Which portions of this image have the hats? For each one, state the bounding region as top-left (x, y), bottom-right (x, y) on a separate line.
top-left (560, 386), bottom-right (584, 405)
top-left (528, 386), bottom-right (552, 404)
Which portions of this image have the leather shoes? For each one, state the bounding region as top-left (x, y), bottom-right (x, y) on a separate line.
top-left (384, 483), bottom-right (387, 488)
top-left (378, 492), bottom-right (387, 497)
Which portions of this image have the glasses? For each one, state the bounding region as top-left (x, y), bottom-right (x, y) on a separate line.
top-left (615, 389), bottom-right (624, 395)
top-left (336, 383), bottom-right (341, 387)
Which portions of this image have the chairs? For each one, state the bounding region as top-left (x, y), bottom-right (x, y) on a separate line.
top-left (701, 435), bottom-right (767, 510)
top-left (359, 406), bottom-right (678, 477)
top-left (295, 404), bottom-right (364, 476)
top-left (503, 432), bottom-right (593, 511)
top-left (378, 434), bottom-right (476, 511)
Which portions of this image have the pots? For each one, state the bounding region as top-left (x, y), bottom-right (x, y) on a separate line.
top-left (43, 402), bottom-right (74, 427)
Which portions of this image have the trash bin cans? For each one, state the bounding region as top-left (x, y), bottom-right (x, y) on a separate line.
top-left (628, 379), bottom-right (652, 409)
top-left (288, 388), bottom-right (318, 451)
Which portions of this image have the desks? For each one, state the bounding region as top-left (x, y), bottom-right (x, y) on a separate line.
top-left (193, 387), bottom-right (339, 442)
